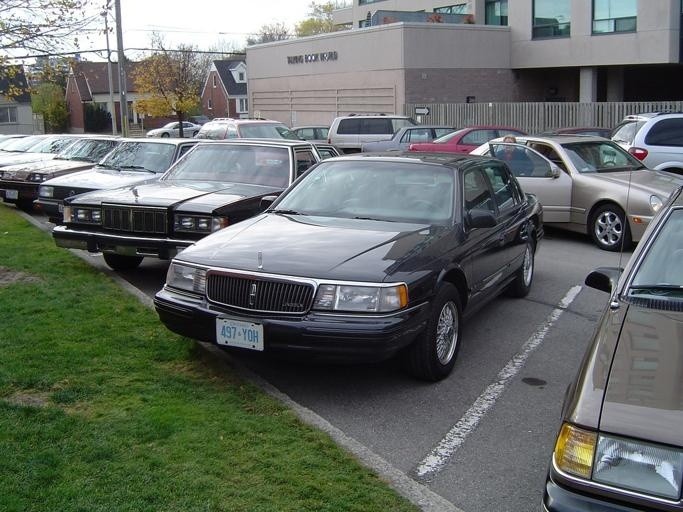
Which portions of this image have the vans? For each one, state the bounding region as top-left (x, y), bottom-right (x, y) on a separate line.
top-left (327, 113), bottom-right (423, 154)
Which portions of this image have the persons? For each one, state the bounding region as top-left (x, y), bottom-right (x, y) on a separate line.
top-left (496, 134), bottom-right (535, 177)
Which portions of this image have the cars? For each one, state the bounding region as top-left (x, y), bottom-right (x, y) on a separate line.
top-left (361, 124), bottom-right (613, 156)
top-left (0, 134), bottom-right (130, 211)
top-left (146, 121), bottom-right (202, 138)
top-left (542, 184), bottom-right (683, 511)
top-left (279, 125), bottom-right (331, 144)
top-left (154, 152), bottom-right (546, 384)
top-left (609, 111), bottom-right (683, 176)
top-left (33, 139), bottom-right (212, 227)
top-left (52, 138), bottom-right (342, 276)
top-left (467, 134), bottom-right (683, 252)
top-left (188, 116), bottom-right (211, 126)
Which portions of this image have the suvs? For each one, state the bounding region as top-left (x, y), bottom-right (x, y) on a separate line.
top-left (212, 118), bottom-right (234, 121)
top-left (192, 117), bottom-right (306, 141)
